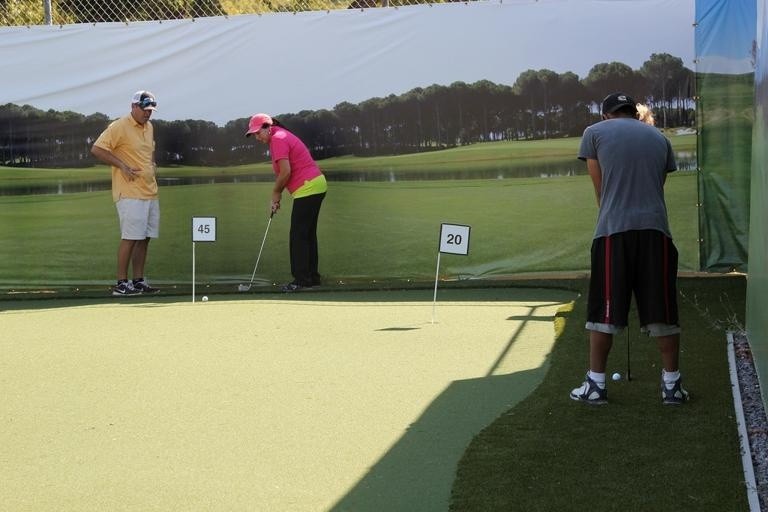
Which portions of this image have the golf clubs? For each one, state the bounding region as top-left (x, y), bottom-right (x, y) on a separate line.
top-left (626, 325), bottom-right (632, 381)
top-left (238, 211), bottom-right (274, 291)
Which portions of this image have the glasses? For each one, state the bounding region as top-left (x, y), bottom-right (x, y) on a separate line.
top-left (144, 102), bottom-right (157, 106)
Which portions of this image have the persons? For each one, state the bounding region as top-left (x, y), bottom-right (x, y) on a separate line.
top-left (569, 91), bottom-right (691, 406)
top-left (91, 90), bottom-right (162, 297)
top-left (244, 114), bottom-right (328, 293)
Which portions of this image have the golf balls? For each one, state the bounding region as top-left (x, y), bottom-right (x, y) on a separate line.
top-left (202, 296), bottom-right (208, 301)
top-left (613, 373), bottom-right (620, 380)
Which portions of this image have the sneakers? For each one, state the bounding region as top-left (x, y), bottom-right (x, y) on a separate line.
top-left (112, 280), bottom-right (160, 297)
top-left (570, 376), bottom-right (608, 405)
top-left (280, 281), bottom-right (321, 291)
top-left (661, 373), bottom-right (690, 405)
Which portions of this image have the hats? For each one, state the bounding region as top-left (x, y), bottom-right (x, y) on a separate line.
top-left (245, 113), bottom-right (273, 138)
top-left (132, 90), bottom-right (158, 112)
top-left (603, 92), bottom-right (636, 120)
